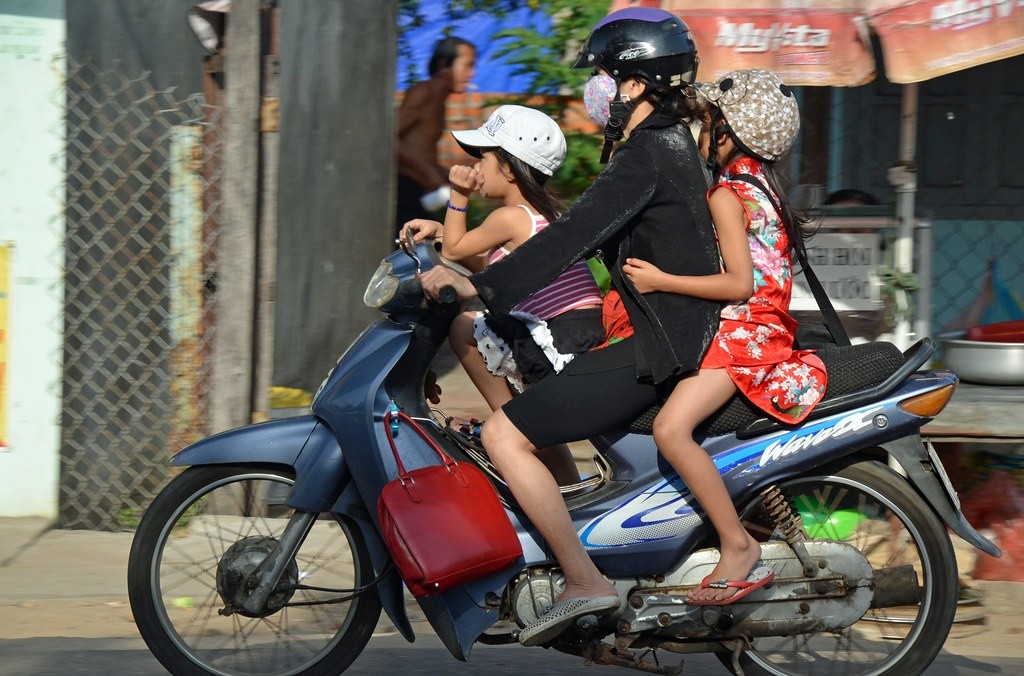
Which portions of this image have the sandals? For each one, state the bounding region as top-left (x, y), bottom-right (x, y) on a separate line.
top-left (445, 416), bottom-right (486, 451)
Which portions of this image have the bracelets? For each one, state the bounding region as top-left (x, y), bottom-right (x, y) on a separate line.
top-left (447, 200), bottom-right (468, 212)
top-left (431, 235), bottom-right (444, 238)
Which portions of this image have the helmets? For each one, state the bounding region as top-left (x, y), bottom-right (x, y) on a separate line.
top-left (698, 67), bottom-right (800, 162)
top-left (568, 7), bottom-right (699, 88)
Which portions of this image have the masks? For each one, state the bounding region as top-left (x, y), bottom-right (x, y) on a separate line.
top-left (583, 75), bottom-right (617, 129)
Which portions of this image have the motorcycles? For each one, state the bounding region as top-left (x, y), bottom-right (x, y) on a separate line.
top-left (128, 223), bottom-right (1001, 676)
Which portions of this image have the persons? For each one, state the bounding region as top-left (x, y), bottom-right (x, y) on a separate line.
top-left (398, 7), bottom-right (828, 647)
top-left (395, 37), bottom-right (482, 256)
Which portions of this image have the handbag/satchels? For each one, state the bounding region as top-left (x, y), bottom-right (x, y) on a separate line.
top-left (795, 319), bottom-right (840, 349)
top-left (376, 411), bottom-right (523, 598)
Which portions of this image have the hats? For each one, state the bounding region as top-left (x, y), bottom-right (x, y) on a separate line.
top-left (451, 104), bottom-right (568, 179)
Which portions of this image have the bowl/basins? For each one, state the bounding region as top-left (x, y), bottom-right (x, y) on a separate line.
top-left (965, 320), bottom-right (1024, 343)
top-left (936, 330), bottom-right (1024, 386)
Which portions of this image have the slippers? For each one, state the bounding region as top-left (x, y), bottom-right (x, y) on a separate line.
top-left (683, 566), bottom-right (774, 606)
top-left (519, 596), bottom-right (621, 646)
